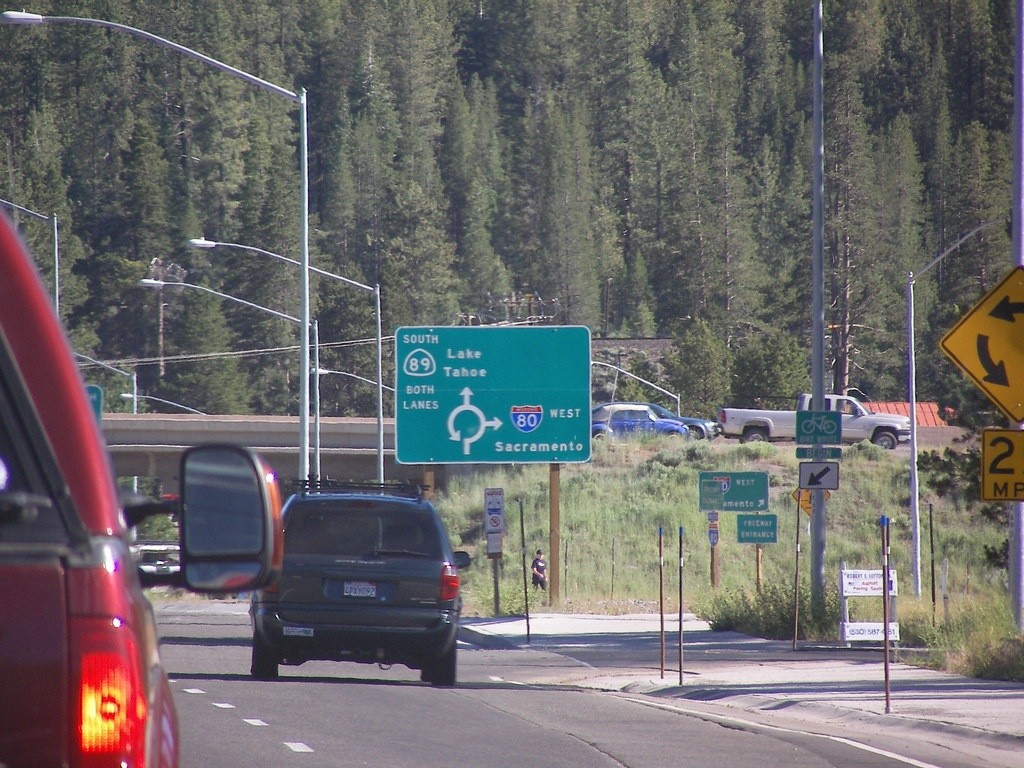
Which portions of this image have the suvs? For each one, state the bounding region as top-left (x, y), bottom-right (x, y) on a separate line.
top-left (253, 477), bottom-right (472, 686)
top-left (0, 211), bottom-right (280, 768)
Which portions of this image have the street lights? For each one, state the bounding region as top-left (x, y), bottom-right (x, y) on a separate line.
top-left (189, 236), bottom-right (385, 490)
top-left (5, 10), bottom-right (311, 494)
top-left (907, 213), bottom-right (1012, 594)
top-left (138, 278), bottom-right (321, 484)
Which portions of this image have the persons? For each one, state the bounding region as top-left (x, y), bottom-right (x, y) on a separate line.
top-left (531, 550), bottom-right (547, 592)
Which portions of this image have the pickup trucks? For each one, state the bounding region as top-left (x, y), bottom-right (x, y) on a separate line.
top-left (719, 393), bottom-right (911, 449)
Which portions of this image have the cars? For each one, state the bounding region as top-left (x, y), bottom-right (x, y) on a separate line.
top-left (591, 405), bottom-right (688, 441)
top-left (592, 401), bottom-right (719, 441)
